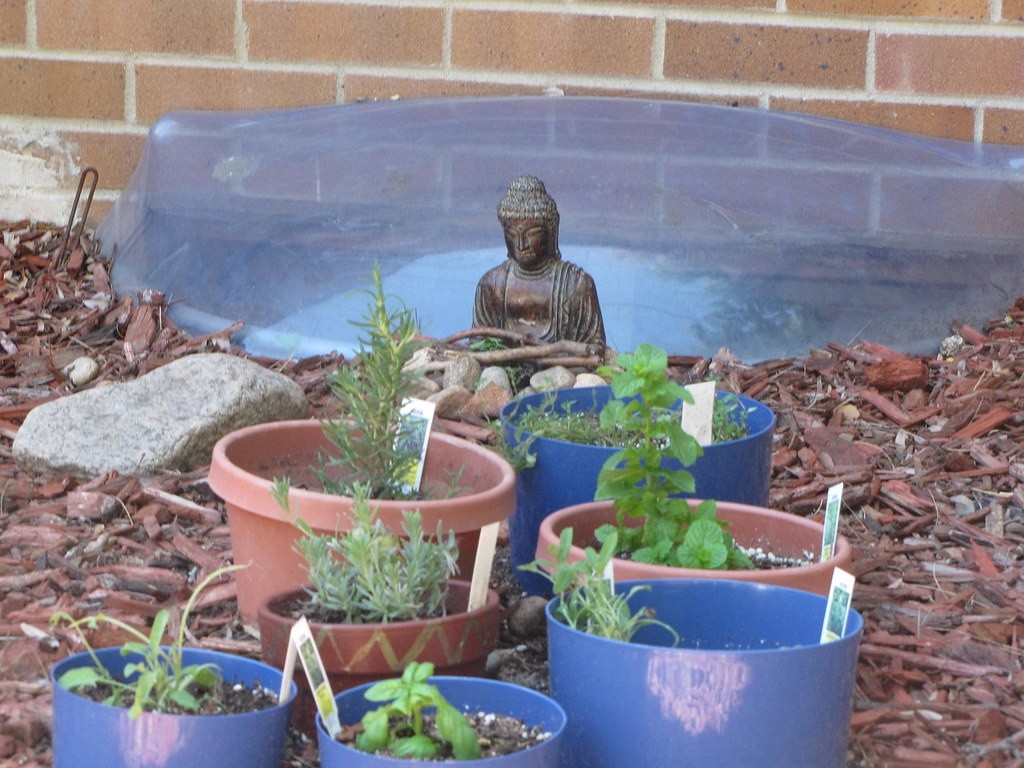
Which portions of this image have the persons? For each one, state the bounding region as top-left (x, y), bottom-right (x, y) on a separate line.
top-left (468, 174), bottom-right (606, 389)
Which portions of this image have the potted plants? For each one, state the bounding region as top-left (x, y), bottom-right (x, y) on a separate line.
top-left (202, 254), bottom-right (518, 633)
top-left (47, 558), bottom-right (301, 768)
top-left (314, 660), bottom-right (568, 768)
top-left (531, 342), bottom-right (852, 597)
top-left (517, 524), bottom-right (864, 768)
top-left (254, 473), bottom-right (508, 739)
top-left (499, 382), bottom-right (779, 601)
